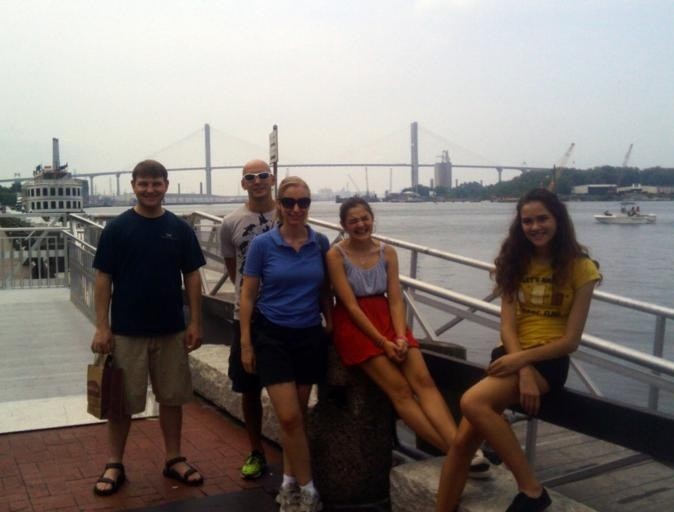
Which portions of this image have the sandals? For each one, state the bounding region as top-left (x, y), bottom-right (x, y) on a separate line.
top-left (93, 462), bottom-right (126, 497)
top-left (163, 456), bottom-right (203, 486)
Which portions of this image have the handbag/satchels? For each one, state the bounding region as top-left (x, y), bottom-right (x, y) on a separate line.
top-left (86, 352), bottom-right (125, 420)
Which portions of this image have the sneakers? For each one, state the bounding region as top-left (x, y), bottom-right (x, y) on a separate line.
top-left (506, 486), bottom-right (552, 512)
top-left (468, 449), bottom-right (493, 480)
top-left (240, 448), bottom-right (267, 480)
top-left (276, 484), bottom-right (323, 512)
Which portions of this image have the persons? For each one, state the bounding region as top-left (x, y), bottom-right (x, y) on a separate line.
top-left (31, 256), bottom-right (56, 279)
top-left (218, 158), bottom-right (282, 483)
top-left (324, 194), bottom-right (495, 481)
top-left (431, 187), bottom-right (602, 512)
top-left (237, 173), bottom-right (351, 511)
top-left (89, 158), bottom-right (207, 497)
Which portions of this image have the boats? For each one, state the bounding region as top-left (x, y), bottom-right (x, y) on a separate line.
top-left (594, 212), bottom-right (657, 225)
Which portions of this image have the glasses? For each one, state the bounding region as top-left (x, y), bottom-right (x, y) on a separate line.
top-left (244, 172), bottom-right (271, 180)
top-left (277, 197), bottom-right (311, 209)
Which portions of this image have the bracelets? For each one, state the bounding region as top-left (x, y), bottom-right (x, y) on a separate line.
top-left (397, 335), bottom-right (409, 344)
